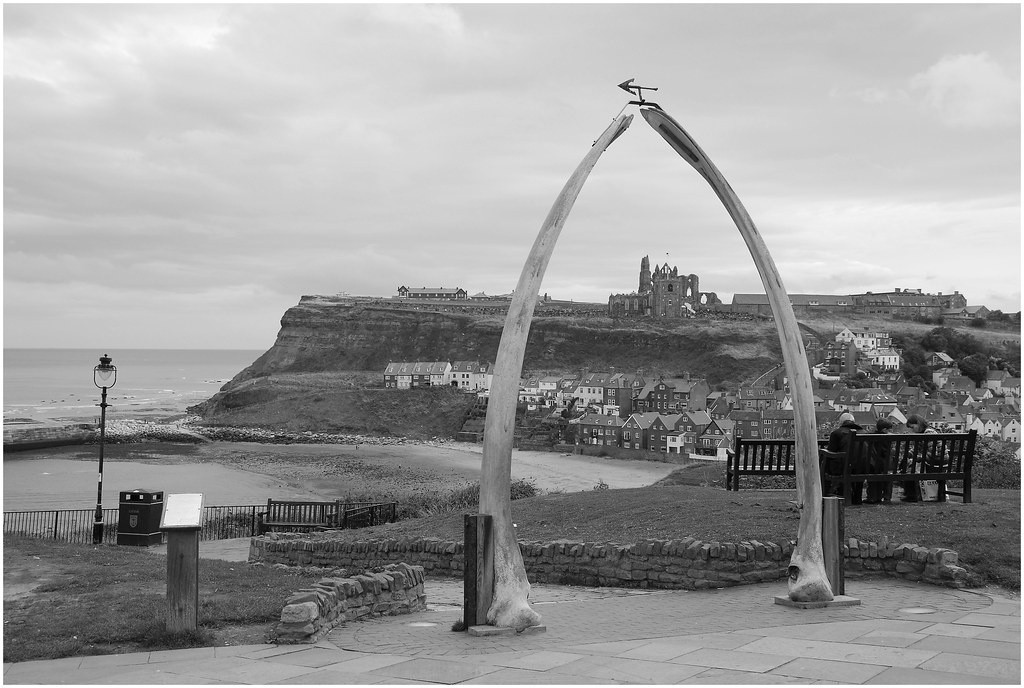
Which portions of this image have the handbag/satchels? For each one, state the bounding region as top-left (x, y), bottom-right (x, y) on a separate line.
top-left (919, 480), bottom-right (949, 502)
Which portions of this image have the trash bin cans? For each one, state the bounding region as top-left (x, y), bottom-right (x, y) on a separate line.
top-left (117, 487), bottom-right (164, 547)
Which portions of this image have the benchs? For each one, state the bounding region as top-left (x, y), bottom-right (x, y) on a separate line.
top-left (257, 497), bottom-right (339, 536)
top-left (723, 433), bottom-right (834, 493)
top-left (817, 428), bottom-right (976, 505)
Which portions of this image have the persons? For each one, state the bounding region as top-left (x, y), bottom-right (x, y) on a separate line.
top-left (867, 416), bottom-right (895, 504)
top-left (824, 420), bottom-right (865, 504)
top-left (899, 415), bottom-right (948, 504)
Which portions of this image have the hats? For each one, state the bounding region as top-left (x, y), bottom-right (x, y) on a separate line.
top-left (877, 417), bottom-right (893, 431)
top-left (839, 413), bottom-right (855, 426)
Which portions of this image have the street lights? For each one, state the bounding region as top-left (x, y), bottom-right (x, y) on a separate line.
top-left (89, 353), bottom-right (117, 544)
